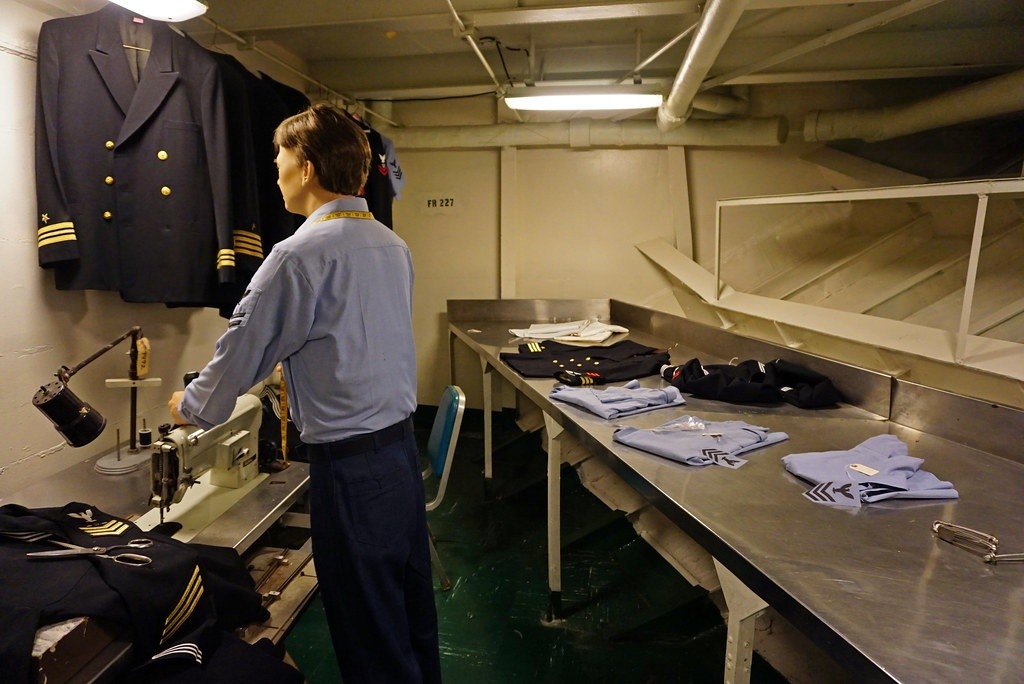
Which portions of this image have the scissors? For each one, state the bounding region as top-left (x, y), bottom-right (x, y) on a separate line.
top-left (26, 538), bottom-right (154, 567)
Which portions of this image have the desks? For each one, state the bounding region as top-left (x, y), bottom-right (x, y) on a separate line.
top-left (445, 296), bottom-right (1024, 684)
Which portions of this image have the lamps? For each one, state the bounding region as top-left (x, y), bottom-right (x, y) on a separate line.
top-left (109, 0), bottom-right (210, 22)
top-left (33, 326), bottom-right (161, 454)
top-left (505, 82), bottom-right (662, 110)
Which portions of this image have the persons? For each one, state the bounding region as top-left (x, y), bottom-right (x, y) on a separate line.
top-left (167, 104), bottom-right (442, 683)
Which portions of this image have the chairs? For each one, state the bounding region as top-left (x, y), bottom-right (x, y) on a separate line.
top-left (419, 386), bottom-right (467, 591)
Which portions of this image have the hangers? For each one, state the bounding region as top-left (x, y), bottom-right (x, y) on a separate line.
top-left (119, 0), bottom-right (154, 53)
top-left (309, 78), bottom-right (374, 132)
